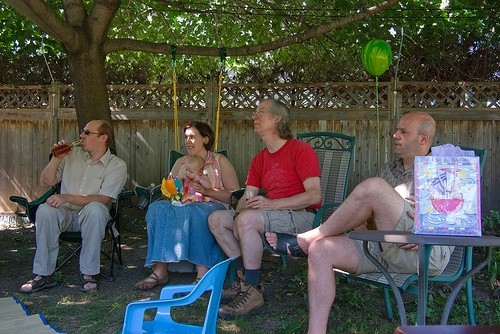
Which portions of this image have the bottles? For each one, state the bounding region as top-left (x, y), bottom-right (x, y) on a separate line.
top-left (51, 140), bottom-right (82, 158)
top-left (138, 182), bottom-right (155, 209)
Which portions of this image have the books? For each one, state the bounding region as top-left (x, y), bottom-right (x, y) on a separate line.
top-left (411, 156), bottom-right (482, 237)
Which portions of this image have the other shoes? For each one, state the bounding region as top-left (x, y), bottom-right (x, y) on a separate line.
top-left (135, 272), bottom-right (170, 291)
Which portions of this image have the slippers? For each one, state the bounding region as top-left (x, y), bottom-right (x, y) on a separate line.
top-left (262, 231), bottom-right (307, 259)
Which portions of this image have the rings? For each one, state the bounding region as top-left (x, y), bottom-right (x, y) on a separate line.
top-left (54, 201), bottom-right (57, 203)
top-left (412, 244), bottom-right (415, 247)
top-left (256, 205), bottom-right (258, 209)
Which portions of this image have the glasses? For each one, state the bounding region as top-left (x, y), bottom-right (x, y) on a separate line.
top-left (80, 128), bottom-right (101, 136)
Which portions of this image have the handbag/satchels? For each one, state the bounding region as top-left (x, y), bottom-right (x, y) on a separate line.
top-left (414, 155), bottom-right (482, 237)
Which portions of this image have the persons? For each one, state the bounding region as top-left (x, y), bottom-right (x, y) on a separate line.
top-left (208, 100), bottom-right (323, 319)
top-left (20, 120), bottom-right (127, 293)
top-left (135, 121), bottom-right (242, 292)
top-left (262, 111), bottom-right (456, 334)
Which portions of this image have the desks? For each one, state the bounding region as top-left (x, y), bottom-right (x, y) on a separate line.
top-left (349, 228), bottom-right (500, 328)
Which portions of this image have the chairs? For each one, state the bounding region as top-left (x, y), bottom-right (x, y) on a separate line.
top-left (9, 132), bottom-right (489, 334)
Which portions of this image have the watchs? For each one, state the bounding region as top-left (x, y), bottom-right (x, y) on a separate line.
top-left (233, 211), bottom-right (240, 220)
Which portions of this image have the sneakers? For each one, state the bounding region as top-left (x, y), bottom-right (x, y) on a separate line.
top-left (200, 267), bottom-right (246, 303)
top-left (218, 281), bottom-right (265, 320)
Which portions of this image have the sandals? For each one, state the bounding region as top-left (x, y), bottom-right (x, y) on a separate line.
top-left (79, 273), bottom-right (98, 291)
top-left (20, 276), bottom-right (57, 292)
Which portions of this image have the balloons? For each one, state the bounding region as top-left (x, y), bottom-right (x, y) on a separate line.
top-left (361, 38), bottom-right (392, 76)
